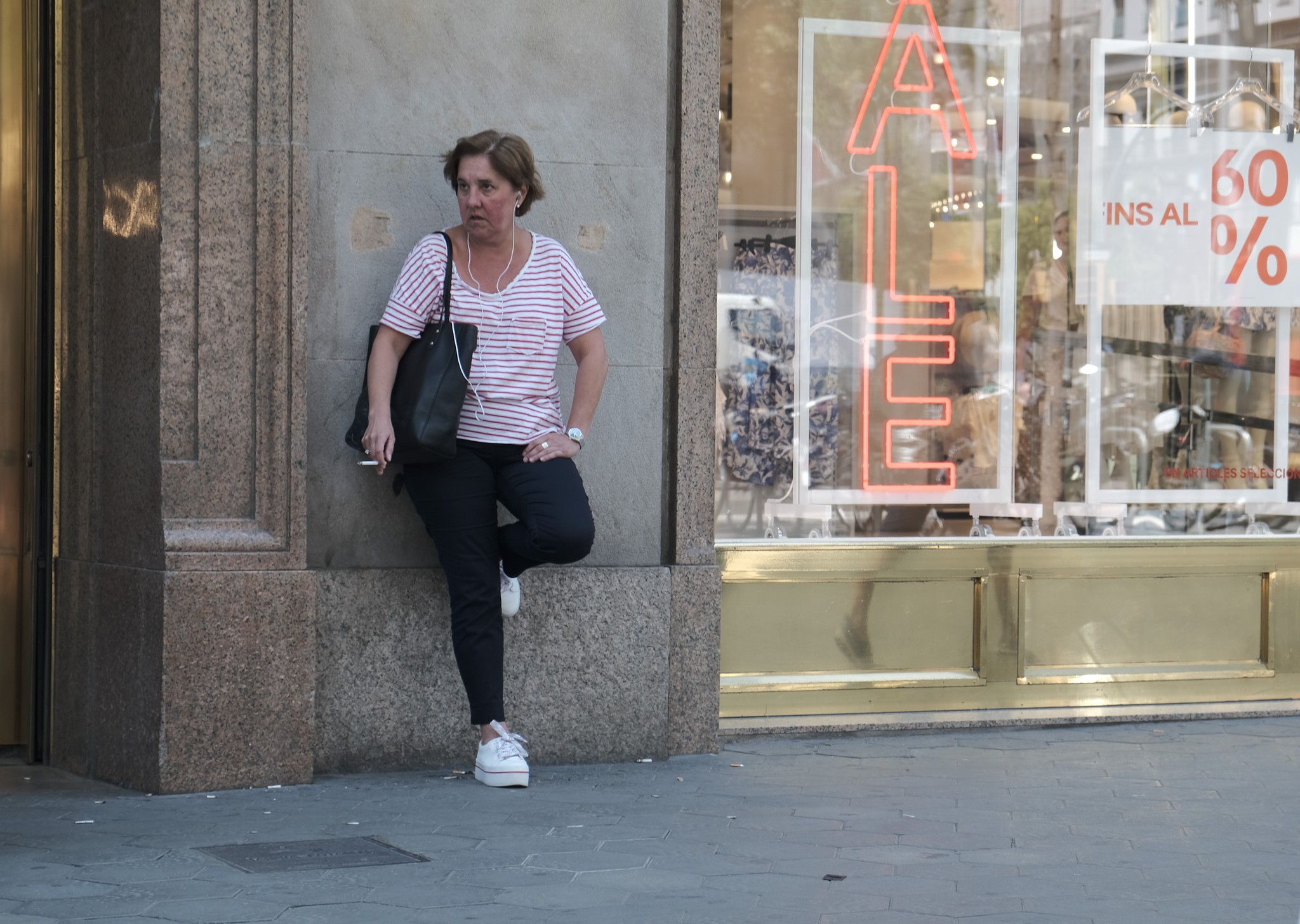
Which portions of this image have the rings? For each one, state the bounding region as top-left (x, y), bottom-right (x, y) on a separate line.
top-left (364, 449), bottom-right (369, 454)
top-left (542, 441), bottom-right (549, 449)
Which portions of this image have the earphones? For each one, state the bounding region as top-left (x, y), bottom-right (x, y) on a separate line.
top-left (517, 191), bottom-right (521, 200)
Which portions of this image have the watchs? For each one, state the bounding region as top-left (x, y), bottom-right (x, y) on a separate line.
top-left (567, 427), bottom-right (586, 449)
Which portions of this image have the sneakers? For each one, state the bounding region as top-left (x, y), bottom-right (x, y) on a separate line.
top-left (499, 561), bottom-right (519, 617)
top-left (475, 719), bottom-right (529, 787)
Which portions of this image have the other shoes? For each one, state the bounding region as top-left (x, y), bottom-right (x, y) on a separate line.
top-left (833, 625), bottom-right (872, 668)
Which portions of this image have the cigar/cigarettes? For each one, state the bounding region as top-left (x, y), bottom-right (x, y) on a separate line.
top-left (636, 758), bottom-right (652, 763)
top-left (266, 784), bottom-right (281, 788)
top-left (357, 461), bottom-right (387, 465)
top-left (452, 770), bottom-right (465, 774)
top-left (730, 763), bottom-right (744, 768)
top-left (75, 820), bottom-right (95, 824)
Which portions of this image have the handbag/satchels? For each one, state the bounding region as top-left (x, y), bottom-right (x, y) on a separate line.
top-left (346, 324), bottom-right (477, 458)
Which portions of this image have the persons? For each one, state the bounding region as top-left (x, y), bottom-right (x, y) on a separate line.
top-left (360, 127), bottom-right (611, 788)
top-left (1086, 80), bottom-right (1278, 493)
top-left (1016, 211), bottom-right (1069, 340)
